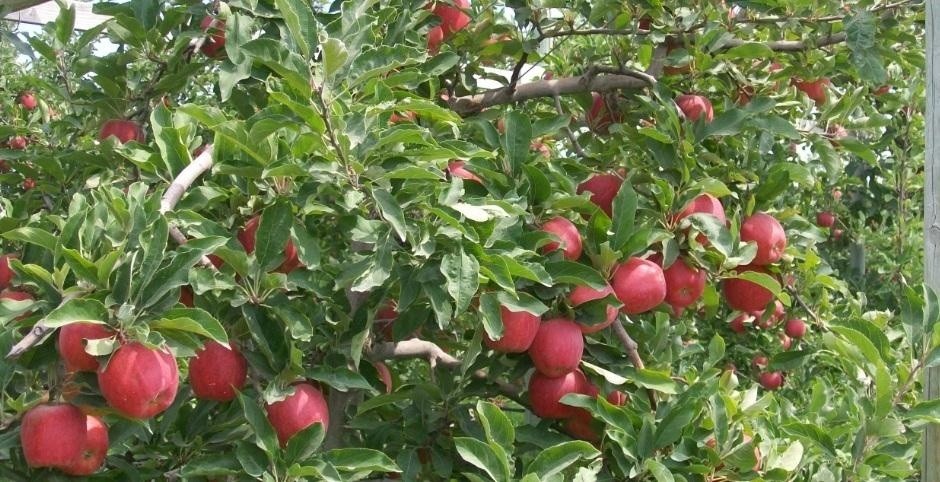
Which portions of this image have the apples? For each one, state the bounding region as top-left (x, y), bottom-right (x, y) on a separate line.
top-left (0, 0), bottom-right (889, 475)
